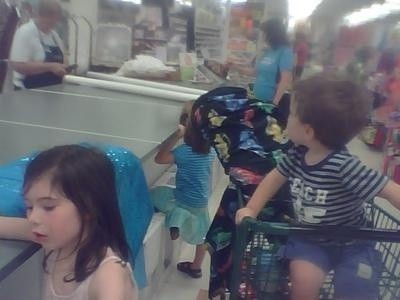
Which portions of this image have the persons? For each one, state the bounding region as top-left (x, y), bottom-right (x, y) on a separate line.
top-left (149, 100), bottom-right (217, 279)
top-left (12, 1), bottom-right (34, 25)
top-left (234, 72), bottom-right (400, 300)
top-left (294, 33), bottom-right (309, 79)
top-left (0, 1), bottom-right (18, 96)
top-left (251, 19), bottom-right (296, 133)
top-left (0, 142), bottom-right (138, 300)
top-left (8, 0), bottom-right (70, 91)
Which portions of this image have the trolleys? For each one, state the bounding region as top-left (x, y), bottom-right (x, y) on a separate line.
top-left (230, 185), bottom-right (400, 300)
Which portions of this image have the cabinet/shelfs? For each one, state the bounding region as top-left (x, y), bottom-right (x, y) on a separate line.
top-left (98, 0), bottom-right (288, 94)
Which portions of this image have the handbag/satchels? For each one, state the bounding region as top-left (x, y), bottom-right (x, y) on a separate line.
top-left (275, 90), bottom-right (291, 130)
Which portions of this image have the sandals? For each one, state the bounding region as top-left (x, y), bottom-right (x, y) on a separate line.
top-left (176, 262), bottom-right (204, 279)
